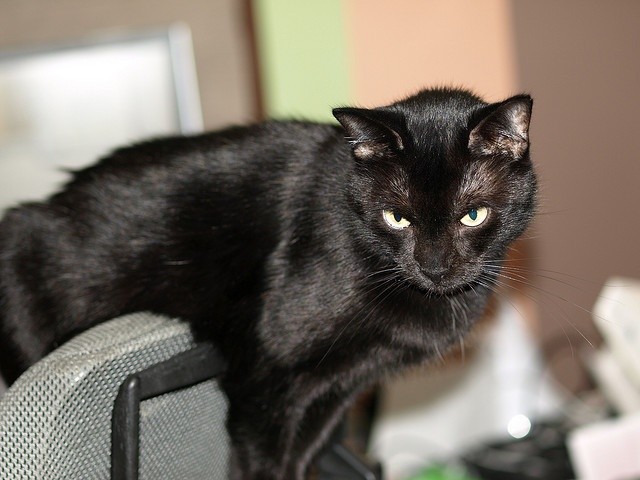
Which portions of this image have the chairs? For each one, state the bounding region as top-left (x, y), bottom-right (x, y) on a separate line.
top-left (0, 309), bottom-right (234, 480)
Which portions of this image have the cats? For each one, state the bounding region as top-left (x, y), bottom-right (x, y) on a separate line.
top-left (0, 84), bottom-right (635, 479)
